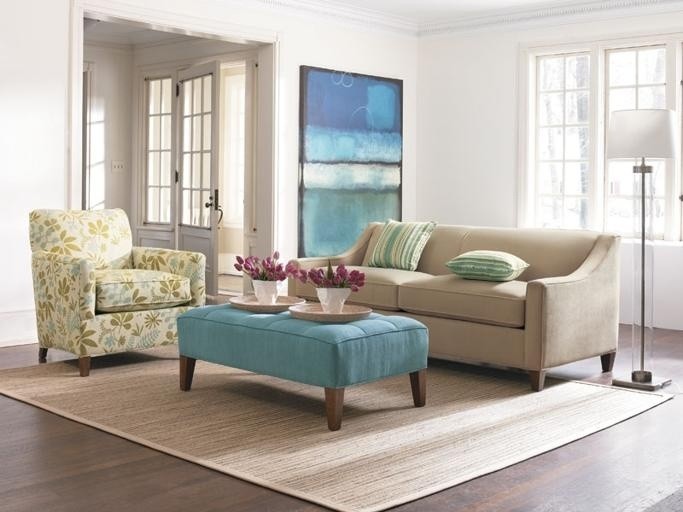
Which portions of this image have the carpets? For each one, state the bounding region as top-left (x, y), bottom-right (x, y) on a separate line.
top-left (0, 355), bottom-right (675, 512)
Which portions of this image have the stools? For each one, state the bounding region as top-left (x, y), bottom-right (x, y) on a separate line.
top-left (177, 302), bottom-right (430, 430)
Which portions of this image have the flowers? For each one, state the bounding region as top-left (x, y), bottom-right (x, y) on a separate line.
top-left (297, 265), bottom-right (368, 292)
top-left (234, 253), bottom-right (301, 282)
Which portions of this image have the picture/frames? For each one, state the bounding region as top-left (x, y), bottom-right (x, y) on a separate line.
top-left (300, 64), bottom-right (402, 260)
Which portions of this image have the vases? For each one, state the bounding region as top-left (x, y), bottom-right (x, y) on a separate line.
top-left (252, 277), bottom-right (281, 303)
top-left (315, 287), bottom-right (352, 312)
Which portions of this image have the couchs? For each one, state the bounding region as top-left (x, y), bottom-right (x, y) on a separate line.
top-left (286, 221), bottom-right (620, 393)
top-left (29, 210), bottom-right (208, 373)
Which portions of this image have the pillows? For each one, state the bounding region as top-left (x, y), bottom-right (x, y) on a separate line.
top-left (367, 217), bottom-right (436, 273)
top-left (445, 249), bottom-right (532, 279)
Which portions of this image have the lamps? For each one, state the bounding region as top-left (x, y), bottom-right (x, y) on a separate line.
top-left (605, 109), bottom-right (679, 392)
